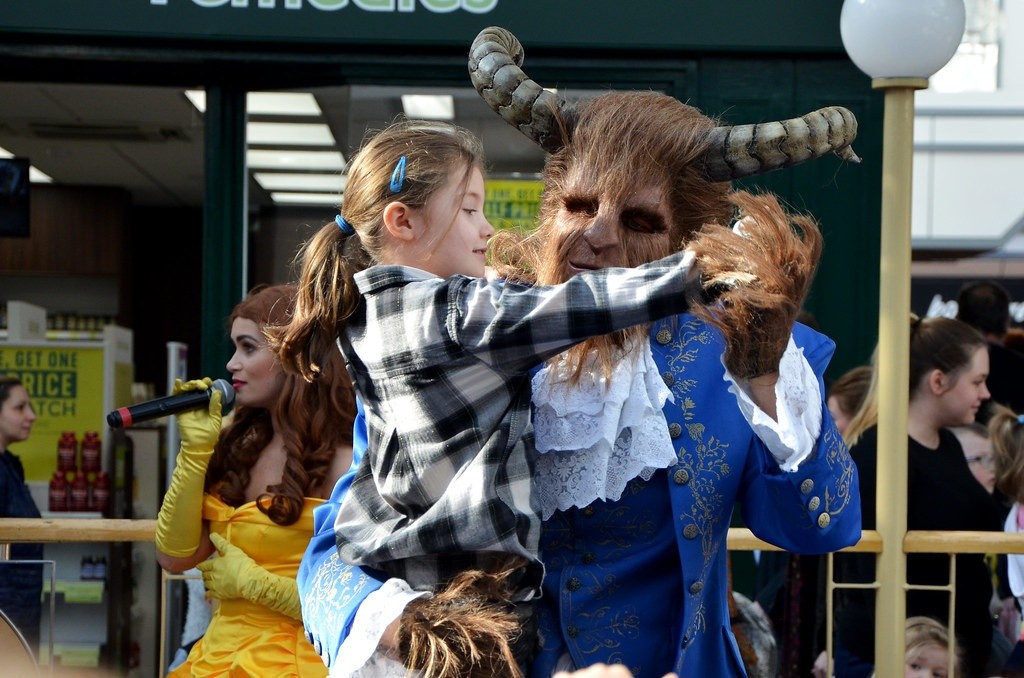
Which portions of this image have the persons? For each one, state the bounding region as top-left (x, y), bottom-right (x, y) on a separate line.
top-left (296, 25), bottom-right (864, 677)
top-left (154, 284), bottom-right (359, 678)
top-left (826, 309), bottom-right (1024, 677)
top-left (0, 376), bottom-right (45, 667)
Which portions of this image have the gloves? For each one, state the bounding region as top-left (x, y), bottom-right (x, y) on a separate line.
top-left (196, 532), bottom-right (302, 622)
top-left (155, 377), bottom-right (222, 558)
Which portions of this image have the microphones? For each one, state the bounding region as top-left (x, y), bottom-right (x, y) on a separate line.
top-left (107, 379), bottom-right (235, 428)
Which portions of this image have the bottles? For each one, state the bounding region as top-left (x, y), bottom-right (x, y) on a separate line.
top-left (81, 555), bottom-right (106, 581)
top-left (48, 430), bottom-right (109, 510)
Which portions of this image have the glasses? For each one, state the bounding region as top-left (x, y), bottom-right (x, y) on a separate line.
top-left (966, 454), bottom-right (996, 467)
